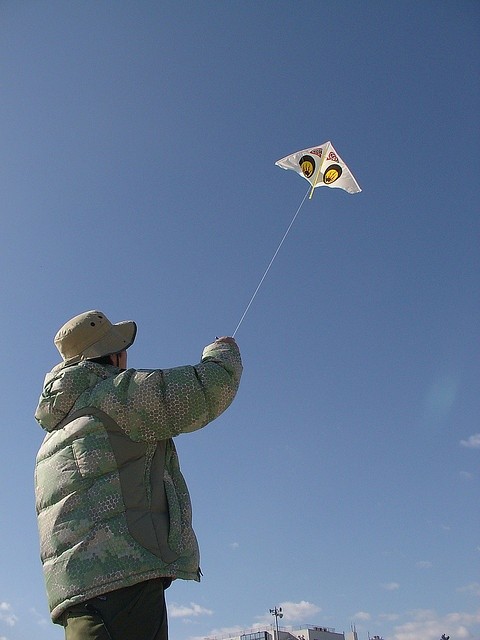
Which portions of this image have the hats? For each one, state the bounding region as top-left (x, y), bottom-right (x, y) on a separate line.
top-left (53, 309), bottom-right (137, 362)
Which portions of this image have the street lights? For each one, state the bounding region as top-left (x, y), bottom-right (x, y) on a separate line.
top-left (270, 607), bottom-right (283, 640)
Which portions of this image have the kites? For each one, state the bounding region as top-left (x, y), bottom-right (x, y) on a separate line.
top-left (273, 139), bottom-right (363, 200)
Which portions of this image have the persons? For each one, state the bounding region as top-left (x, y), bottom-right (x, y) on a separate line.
top-left (32, 308), bottom-right (242, 640)
